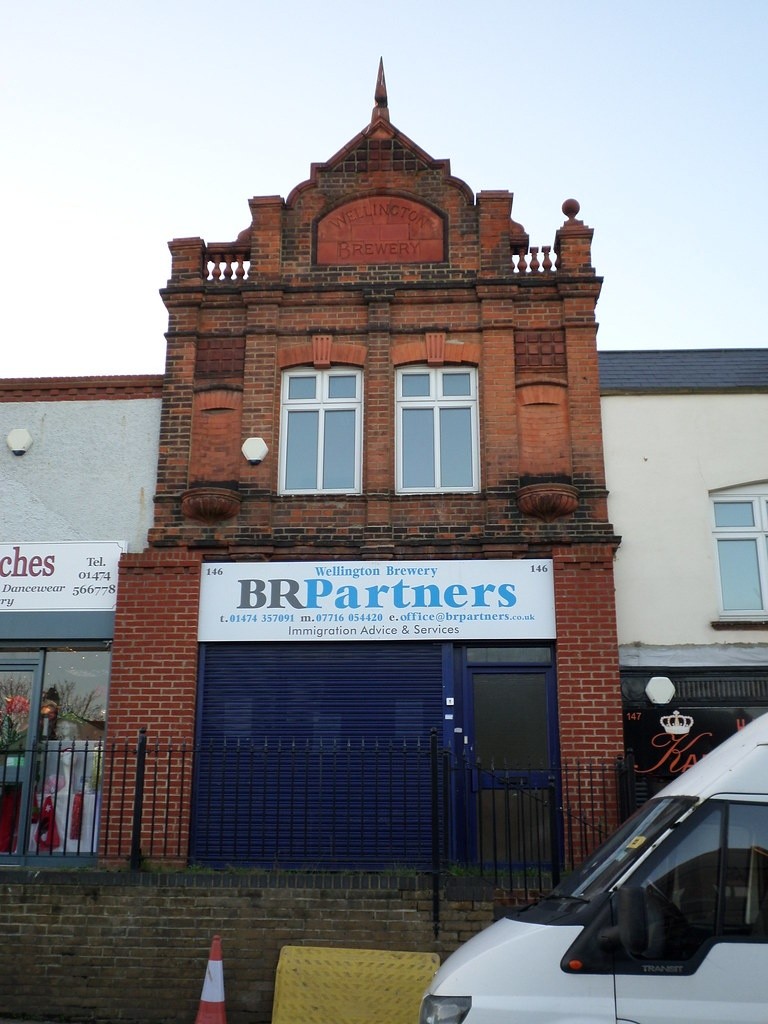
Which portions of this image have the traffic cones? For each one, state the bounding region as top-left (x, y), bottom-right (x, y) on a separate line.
top-left (194, 935), bottom-right (227, 1024)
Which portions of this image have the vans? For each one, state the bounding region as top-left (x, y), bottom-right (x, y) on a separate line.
top-left (420, 712), bottom-right (768, 1024)
